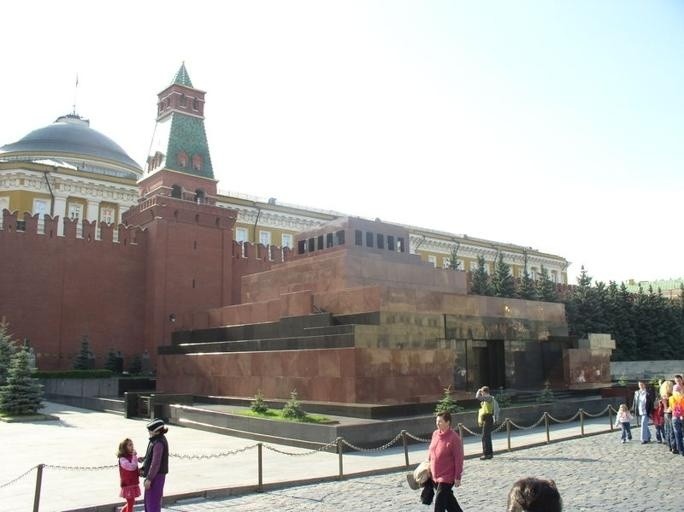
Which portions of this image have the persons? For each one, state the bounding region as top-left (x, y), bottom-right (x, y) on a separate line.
top-left (139, 418), bottom-right (168, 512)
top-left (632, 381), bottom-right (655, 444)
top-left (476, 386), bottom-right (493, 460)
top-left (116, 438), bottom-right (143, 512)
top-left (427, 412), bottom-right (464, 512)
top-left (615, 404), bottom-right (633, 443)
top-left (652, 375), bottom-right (684, 456)
top-left (506, 478), bottom-right (563, 512)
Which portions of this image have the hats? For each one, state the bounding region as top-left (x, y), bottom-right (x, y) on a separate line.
top-left (147, 418), bottom-right (164, 433)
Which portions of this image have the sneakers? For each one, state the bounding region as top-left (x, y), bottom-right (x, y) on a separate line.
top-left (622, 439), bottom-right (631, 443)
top-left (641, 437), bottom-right (650, 443)
top-left (480, 453), bottom-right (493, 460)
